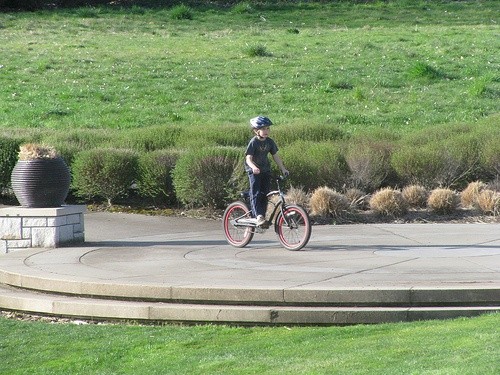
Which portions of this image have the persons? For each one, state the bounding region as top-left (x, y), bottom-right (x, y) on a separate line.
top-left (244, 116), bottom-right (290, 229)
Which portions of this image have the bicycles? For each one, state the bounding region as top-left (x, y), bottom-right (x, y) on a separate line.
top-left (222, 172), bottom-right (312, 251)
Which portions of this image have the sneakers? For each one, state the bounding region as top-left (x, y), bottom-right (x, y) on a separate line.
top-left (256, 216), bottom-right (269, 226)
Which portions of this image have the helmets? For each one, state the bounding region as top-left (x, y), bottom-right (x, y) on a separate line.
top-left (250, 116), bottom-right (274, 129)
